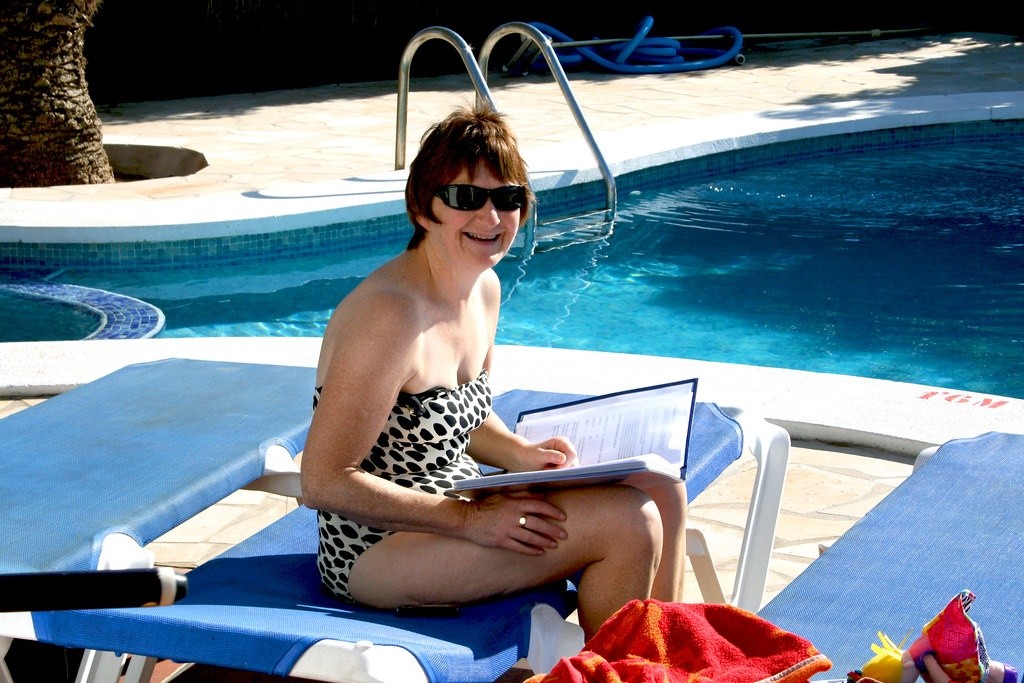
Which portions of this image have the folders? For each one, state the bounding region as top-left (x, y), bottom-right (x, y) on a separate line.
top-left (445, 376), bottom-right (699, 494)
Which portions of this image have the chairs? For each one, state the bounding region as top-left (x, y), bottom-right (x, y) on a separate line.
top-left (0, 358), bottom-right (1024, 683)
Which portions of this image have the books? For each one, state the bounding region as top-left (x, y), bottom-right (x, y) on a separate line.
top-left (449, 377), bottom-right (700, 505)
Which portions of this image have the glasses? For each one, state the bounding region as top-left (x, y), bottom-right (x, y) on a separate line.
top-left (434, 184), bottom-right (526, 212)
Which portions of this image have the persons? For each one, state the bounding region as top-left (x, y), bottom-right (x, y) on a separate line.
top-left (300, 107), bottom-right (689, 650)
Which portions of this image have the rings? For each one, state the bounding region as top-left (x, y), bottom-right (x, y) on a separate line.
top-left (518, 513), bottom-right (528, 527)
